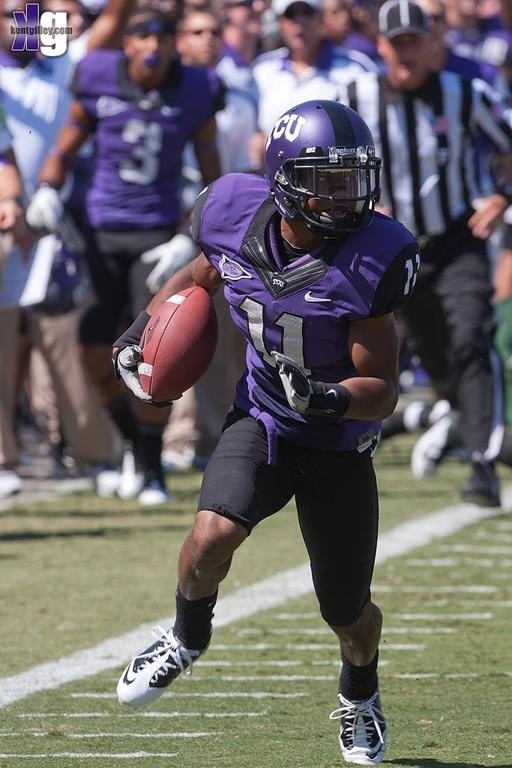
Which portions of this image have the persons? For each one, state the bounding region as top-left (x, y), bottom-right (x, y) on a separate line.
top-left (111, 99), bottom-right (423, 766)
top-left (0, 0), bottom-right (509, 517)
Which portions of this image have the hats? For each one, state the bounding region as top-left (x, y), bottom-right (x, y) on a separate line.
top-left (273, 0), bottom-right (320, 18)
top-left (379, 1), bottom-right (429, 40)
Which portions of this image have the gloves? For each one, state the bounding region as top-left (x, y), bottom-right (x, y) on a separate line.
top-left (111, 344), bottom-right (173, 408)
top-left (271, 350), bottom-right (322, 415)
top-left (141, 234), bottom-right (199, 295)
top-left (25, 187), bottom-right (64, 230)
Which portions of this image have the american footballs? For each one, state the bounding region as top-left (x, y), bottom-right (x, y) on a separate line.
top-left (138, 285), bottom-right (218, 402)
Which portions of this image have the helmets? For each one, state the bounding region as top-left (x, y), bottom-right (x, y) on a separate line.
top-left (264, 100), bottom-right (382, 240)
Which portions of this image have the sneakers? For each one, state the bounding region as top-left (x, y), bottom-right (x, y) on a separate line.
top-left (461, 474), bottom-right (501, 508)
top-left (329, 663), bottom-right (387, 765)
top-left (139, 465), bottom-right (170, 503)
top-left (117, 626), bottom-right (213, 707)
